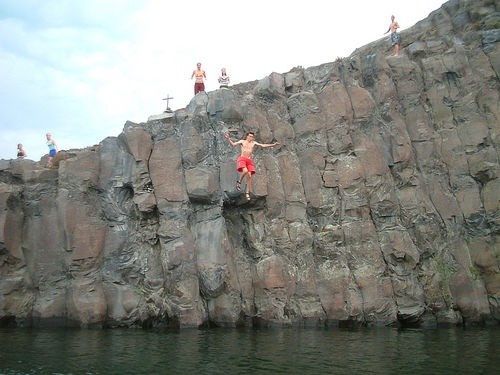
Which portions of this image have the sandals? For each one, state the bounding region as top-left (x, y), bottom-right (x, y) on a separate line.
top-left (247, 193), bottom-right (251, 201)
top-left (235, 181), bottom-right (241, 190)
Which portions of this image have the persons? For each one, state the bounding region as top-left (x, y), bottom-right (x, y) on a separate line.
top-left (191, 63), bottom-right (206, 95)
top-left (46, 134), bottom-right (58, 161)
top-left (17, 144), bottom-right (26, 159)
top-left (384, 16), bottom-right (400, 57)
top-left (223, 131), bottom-right (280, 201)
top-left (218, 68), bottom-right (230, 88)
